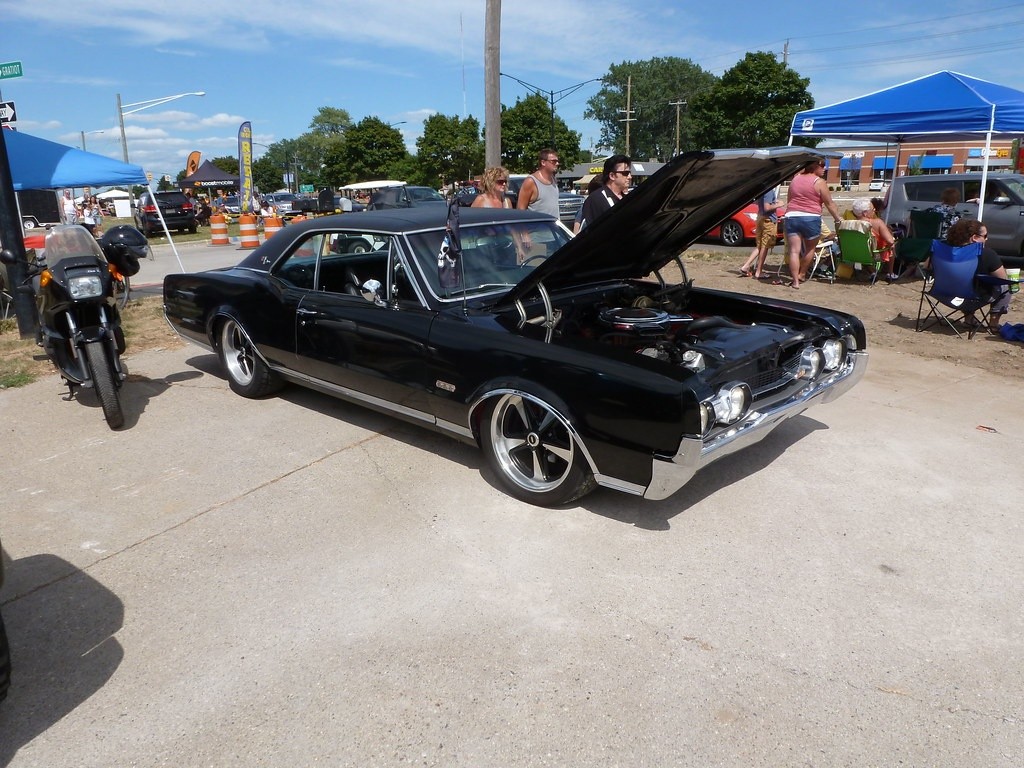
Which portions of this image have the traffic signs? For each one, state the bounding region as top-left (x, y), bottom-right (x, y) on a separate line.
top-left (0, 101), bottom-right (18, 124)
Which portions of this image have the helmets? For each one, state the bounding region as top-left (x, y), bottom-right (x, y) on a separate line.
top-left (101, 225), bottom-right (149, 277)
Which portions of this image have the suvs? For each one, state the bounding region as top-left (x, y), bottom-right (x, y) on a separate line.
top-left (868, 179), bottom-right (884, 191)
top-left (504, 173), bottom-right (587, 232)
top-left (365, 185), bottom-right (447, 212)
top-left (879, 172), bottom-right (1024, 262)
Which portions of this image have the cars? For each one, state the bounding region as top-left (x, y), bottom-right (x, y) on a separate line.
top-left (693, 202), bottom-right (786, 248)
top-left (215, 194), bottom-right (240, 215)
top-left (263, 194), bottom-right (303, 217)
top-left (884, 180), bottom-right (892, 187)
top-left (333, 197), bottom-right (364, 214)
top-left (162, 145), bottom-right (870, 508)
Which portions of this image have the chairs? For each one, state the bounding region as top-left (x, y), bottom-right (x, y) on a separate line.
top-left (779, 218), bottom-right (836, 281)
top-left (839, 219), bottom-right (897, 286)
top-left (901, 209), bottom-right (945, 283)
top-left (916, 239), bottom-right (1024, 340)
top-left (458, 247), bottom-right (506, 290)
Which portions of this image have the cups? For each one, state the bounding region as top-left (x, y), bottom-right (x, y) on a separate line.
top-left (1006, 268), bottom-right (1021, 292)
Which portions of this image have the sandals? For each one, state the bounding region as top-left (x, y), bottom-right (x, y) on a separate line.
top-left (962, 318), bottom-right (986, 333)
top-left (986, 324), bottom-right (1002, 335)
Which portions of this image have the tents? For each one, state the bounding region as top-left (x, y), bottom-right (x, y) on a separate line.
top-left (0, 127), bottom-right (186, 274)
top-left (173, 159), bottom-right (240, 212)
top-left (775, 68), bottom-right (1024, 223)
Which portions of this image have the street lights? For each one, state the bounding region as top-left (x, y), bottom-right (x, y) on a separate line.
top-left (81, 130), bottom-right (104, 152)
top-left (499, 73), bottom-right (605, 151)
top-left (116, 91), bottom-right (206, 218)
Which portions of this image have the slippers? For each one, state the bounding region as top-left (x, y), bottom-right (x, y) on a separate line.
top-left (789, 282), bottom-right (793, 287)
top-left (739, 269), bottom-right (752, 276)
top-left (754, 273), bottom-right (770, 279)
top-left (798, 279), bottom-right (804, 284)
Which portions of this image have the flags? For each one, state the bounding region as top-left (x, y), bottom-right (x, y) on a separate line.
top-left (187, 151), bottom-right (201, 177)
top-left (238, 121), bottom-right (253, 213)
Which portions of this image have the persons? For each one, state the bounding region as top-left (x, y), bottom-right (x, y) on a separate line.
top-left (740, 189), bottom-right (784, 278)
top-left (252, 191), bottom-right (273, 223)
top-left (573, 154), bottom-right (632, 235)
top-left (471, 168), bottom-right (525, 262)
top-left (515, 148), bottom-right (561, 265)
top-left (929, 187), bottom-right (962, 238)
top-left (852, 198), bottom-right (899, 280)
top-left (186, 192), bottom-right (228, 227)
top-left (784, 156), bottom-right (843, 289)
top-left (923, 218), bottom-right (1011, 333)
top-left (61, 190), bottom-right (104, 236)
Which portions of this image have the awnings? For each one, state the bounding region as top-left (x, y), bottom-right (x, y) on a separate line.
top-left (965, 158), bottom-right (1013, 167)
top-left (907, 155), bottom-right (954, 168)
top-left (839, 158), bottom-right (862, 169)
top-left (872, 156), bottom-right (897, 169)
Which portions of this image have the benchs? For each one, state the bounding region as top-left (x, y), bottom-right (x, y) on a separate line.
top-left (287, 249), bottom-right (390, 293)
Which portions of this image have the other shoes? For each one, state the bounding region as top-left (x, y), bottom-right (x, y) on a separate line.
top-left (886, 273), bottom-right (899, 279)
top-left (871, 273), bottom-right (877, 282)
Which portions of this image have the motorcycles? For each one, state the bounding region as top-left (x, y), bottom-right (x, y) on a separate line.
top-left (0, 223), bottom-right (127, 430)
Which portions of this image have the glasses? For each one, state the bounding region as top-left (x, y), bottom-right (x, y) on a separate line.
top-left (817, 163), bottom-right (825, 168)
top-left (613, 171), bottom-right (631, 176)
top-left (970, 233), bottom-right (987, 239)
top-left (495, 180), bottom-right (508, 185)
top-left (545, 160), bottom-right (559, 164)
